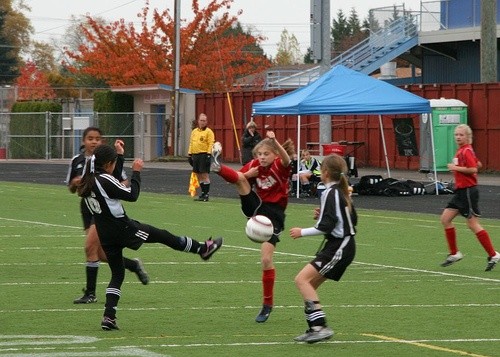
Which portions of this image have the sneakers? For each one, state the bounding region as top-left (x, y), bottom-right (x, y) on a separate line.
top-left (305, 324), bottom-right (334, 341)
top-left (440, 250), bottom-right (462, 266)
top-left (209, 142), bottom-right (222, 174)
top-left (133, 258), bottom-right (148, 285)
top-left (256, 305), bottom-right (272, 322)
top-left (293, 329), bottom-right (310, 341)
top-left (484, 250), bottom-right (500, 271)
top-left (101, 317), bottom-right (120, 330)
top-left (74, 288), bottom-right (97, 304)
top-left (201, 236), bottom-right (222, 261)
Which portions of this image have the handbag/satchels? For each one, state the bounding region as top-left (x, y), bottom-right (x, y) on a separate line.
top-left (355, 175), bottom-right (427, 196)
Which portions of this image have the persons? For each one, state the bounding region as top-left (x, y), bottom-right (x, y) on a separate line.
top-left (440, 124), bottom-right (500, 273)
top-left (290, 151), bottom-right (332, 198)
top-left (210, 130), bottom-right (289, 322)
top-left (73, 140), bottom-right (222, 331)
top-left (289, 155), bottom-right (359, 343)
top-left (187, 113), bottom-right (221, 202)
top-left (241, 122), bottom-right (260, 166)
top-left (64, 128), bottom-right (149, 304)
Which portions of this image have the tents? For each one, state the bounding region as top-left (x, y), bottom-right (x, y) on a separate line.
top-left (249, 62), bottom-right (440, 196)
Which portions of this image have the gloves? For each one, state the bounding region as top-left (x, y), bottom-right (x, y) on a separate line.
top-left (188, 157), bottom-right (193, 166)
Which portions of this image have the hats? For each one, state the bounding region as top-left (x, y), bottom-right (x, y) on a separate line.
top-left (246, 121), bottom-right (257, 130)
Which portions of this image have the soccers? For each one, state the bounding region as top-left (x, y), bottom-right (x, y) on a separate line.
top-left (243, 213), bottom-right (273, 243)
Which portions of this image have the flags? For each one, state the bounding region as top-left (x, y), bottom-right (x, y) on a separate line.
top-left (188, 171), bottom-right (200, 198)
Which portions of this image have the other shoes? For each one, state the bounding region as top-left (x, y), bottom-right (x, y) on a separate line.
top-left (194, 197), bottom-right (208, 202)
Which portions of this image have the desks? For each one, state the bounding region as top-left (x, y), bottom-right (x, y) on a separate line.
top-left (307, 141), bottom-right (366, 155)
